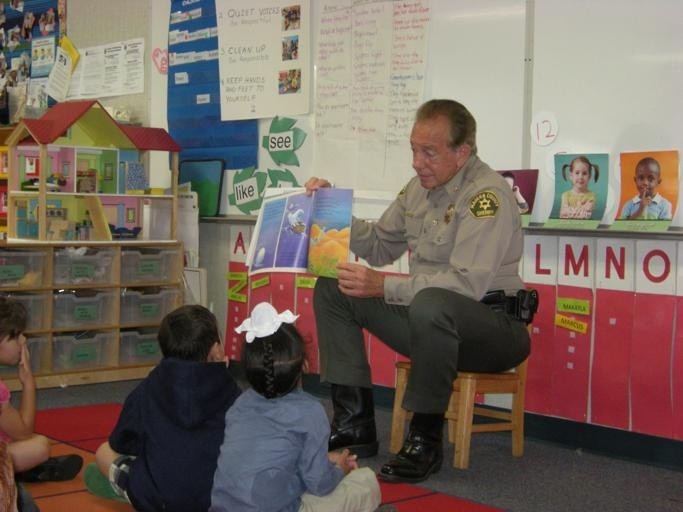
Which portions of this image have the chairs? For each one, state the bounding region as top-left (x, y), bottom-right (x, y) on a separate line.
top-left (388, 321), bottom-right (530, 471)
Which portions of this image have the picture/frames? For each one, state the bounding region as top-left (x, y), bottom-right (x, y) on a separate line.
top-left (178, 159), bottom-right (224, 217)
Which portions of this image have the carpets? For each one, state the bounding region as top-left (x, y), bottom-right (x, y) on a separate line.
top-left (0, 402), bottom-right (511, 512)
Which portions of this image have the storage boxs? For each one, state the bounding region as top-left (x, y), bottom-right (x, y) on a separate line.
top-left (0, 294), bottom-right (46, 330)
top-left (119, 248), bottom-right (180, 286)
top-left (53, 247), bottom-right (117, 286)
top-left (51, 334), bottom-right (116, 374)
top-left (0, 250), bottom-right (49, 291)
top-left (119, 290), bottom-right (176, 324)
top-left (0, 338), bottom-right (47, 374)
top-left (52, 291), bottom-right (115, 328)
top-left (118, 329), bottom-right (163, 370)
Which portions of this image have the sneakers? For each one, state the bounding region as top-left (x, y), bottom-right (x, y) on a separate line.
top-left (82, 462), bottom-right (129, 505)
top-left (14, 453), bottom-right (83, 483)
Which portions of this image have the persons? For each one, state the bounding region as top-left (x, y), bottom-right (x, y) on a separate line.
top-left (559, 157), bottom-right (600, 220)
top-left (0, 295), bottom-right (85, 484)
top-left (0, 441), bottom-right (39, 512)
top-left (618, 157), bottom-right (673, 220)
top-left (501, 171), bottom-right (530, 215)
top-left (82, 304), bottom-right (244, 512)
top-left (312, 98), bottom-right (538, 482)
top-left (206, 301), bottom-right (397, 512)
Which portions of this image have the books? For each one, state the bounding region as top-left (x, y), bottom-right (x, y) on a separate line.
top-left (244, 187), bottom-right (353, 280)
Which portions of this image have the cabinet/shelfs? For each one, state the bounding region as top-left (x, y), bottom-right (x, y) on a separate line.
top-left (0, 238), bottom-right (185, 392)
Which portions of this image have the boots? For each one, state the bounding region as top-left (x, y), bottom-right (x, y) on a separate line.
top-left (327, 383), bottom-right (379, 459)
top-left (376, 411), bottom-right (442, 484)
